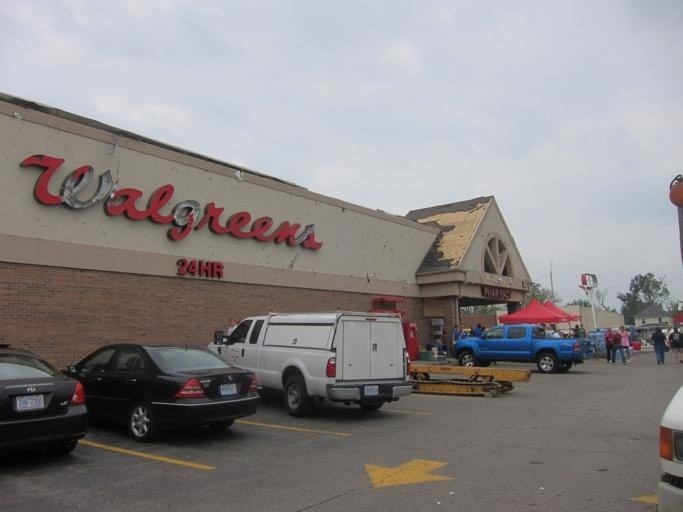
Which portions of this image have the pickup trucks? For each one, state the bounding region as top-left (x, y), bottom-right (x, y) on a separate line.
top-left (452, 323), bottom-right (593, 373)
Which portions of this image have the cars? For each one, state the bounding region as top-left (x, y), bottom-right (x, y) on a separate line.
top-left (58, 342), bottom-right (260, 443)
top-left (0, 342), bottom-right (88, 462)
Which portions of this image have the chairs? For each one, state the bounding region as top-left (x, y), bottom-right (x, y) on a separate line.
top-left (119, 357), bottom-right (142, 371)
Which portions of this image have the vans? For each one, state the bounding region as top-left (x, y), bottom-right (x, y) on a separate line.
top-left (206, 309), bottom-right (411, 418)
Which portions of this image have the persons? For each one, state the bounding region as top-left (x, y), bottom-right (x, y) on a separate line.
top-left (527, 322), bottom-right (565, 338)
top-left (571, 323), bottom-right (586, 339)
top-left (602, 326), bottom-right (631, 367)
top-left (648, 326), bottom-right (683, 366)
top-left (227, 319), bottom-right (238, 336)
top-left (453, 323), bottom-right (486, 345)
